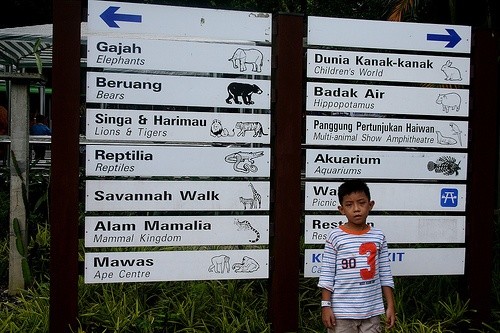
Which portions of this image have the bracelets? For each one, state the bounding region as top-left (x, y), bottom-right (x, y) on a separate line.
top-left (320, 300), bottom-right (331, 307)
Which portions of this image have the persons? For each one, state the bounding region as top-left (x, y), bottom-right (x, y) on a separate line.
top-left (28, 115), bottom-right (51, 160)
top-left (316, 179), bottom-right (395, 333)
